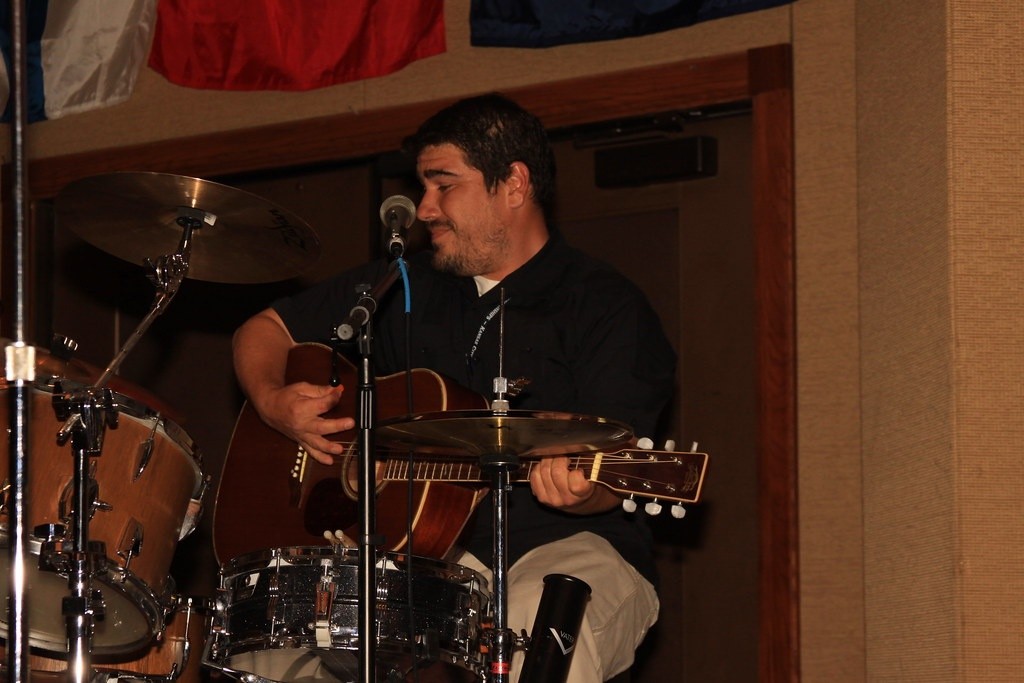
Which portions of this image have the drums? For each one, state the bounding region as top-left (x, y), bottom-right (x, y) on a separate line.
top-left (1, 370), bottom-right (210, 668)
top-left (210, 545), bottom-right (497, 681)
top-left (12, 592), bottom-right (209, 683)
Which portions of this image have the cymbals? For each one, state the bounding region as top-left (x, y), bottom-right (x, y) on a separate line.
top-left (361, 406), bottom-right (637, 461)
top-left (52, 168), bottom-right (325, 285)
top-left (0, 335), bottom-right (178, 420)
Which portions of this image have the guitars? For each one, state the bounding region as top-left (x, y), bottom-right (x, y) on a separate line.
top-left (208, 340), bottom-right (714, 569)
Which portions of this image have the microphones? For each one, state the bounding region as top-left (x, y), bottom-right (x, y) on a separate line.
top-left (378, 194), bottom-right (418, 257)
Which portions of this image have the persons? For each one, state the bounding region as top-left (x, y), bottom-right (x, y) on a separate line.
top-left (229, 92), bottom-right (680, 683)
